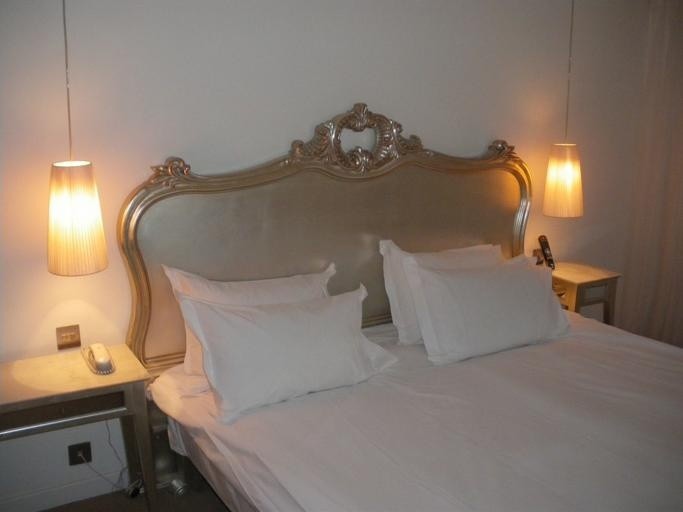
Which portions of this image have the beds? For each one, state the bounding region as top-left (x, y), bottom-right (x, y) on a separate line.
top-left (119, 104), bottom-right (682, 512)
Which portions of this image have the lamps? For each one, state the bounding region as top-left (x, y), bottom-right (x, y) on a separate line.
top-left (541, 2), bottom-right (584, 220)
top-left (44, 2), bottom-right (109, 275)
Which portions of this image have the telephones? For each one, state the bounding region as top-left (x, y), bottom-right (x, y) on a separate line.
top-left (86, 343), bottom-right (113, 372)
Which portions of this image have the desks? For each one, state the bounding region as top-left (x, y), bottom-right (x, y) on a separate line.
top-left (0, 342), bottom-right (166, 510)
top-left (550, 261), bottom-right (621, 328)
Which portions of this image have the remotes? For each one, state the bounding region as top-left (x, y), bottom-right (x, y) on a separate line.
top-left (538, 235), bottom-right (554, 269)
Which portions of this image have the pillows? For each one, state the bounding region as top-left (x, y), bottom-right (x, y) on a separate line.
top-left (159, 256), bottom-right (335, 400)
top-left (376, 237), bottom-right (505, 348)
top-left (401, 253), bottom-right (570, 362)
top-left (180, 285), bottom-right (396, 423)
